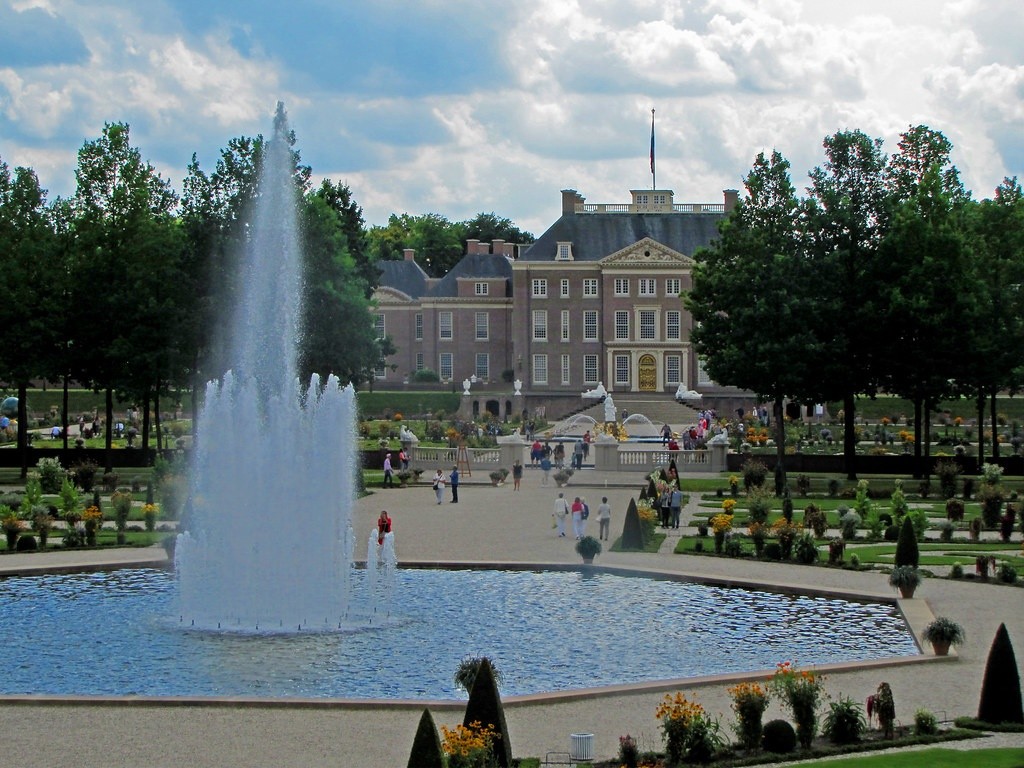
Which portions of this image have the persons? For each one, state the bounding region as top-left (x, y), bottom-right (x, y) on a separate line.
top-left (525, 420), bottom-right (590, 487)
top-left (513, 459), bottom-right (522, 491)
top-left (571, 496), bottom-right (589, 540)
top-left (378, 511), bottom-right (392, 546)
top-left (597, 382), bottom-right (606, 395)
top-left (669, 436), bottom-right (679, 462)
top-left (660, 423), bottom-right (671, 447)
top-left (661, 485), bottom-right (670, 529)
top-left (52, 425), bottom-right (71, 438)
top-left (79, 416), bottom-right (85, 435)
top-left (604, 394), bottom-right (614, 406)
top-left (399, 447), bottom-right (410, 473)
top-left (0, 415), bottom-right (9, 430)
top-left (670, 484), bottom-right (683, 530)
top-left (551, 493), bottom-right (569, 537)
top-left (450, 465), bottom-right (458, 504)
top-left (383, 454), bottom-right (394, 488)
top-left (676, 382), bottom-right (687, 399)
top-left (92, 415), bottom-right (101, 435)
top-left (734, 406), bottom-right (744, 419)
top-left (597, 497), bottom-right (612, 540)
top-left (433, 470), bottom-right (445, 504)
top-left (683, 408), bottom-right (728, 449)
top-left (752, 406), bottom-right (767, 425)
top-left (622, 409), bottom-right (628, 421)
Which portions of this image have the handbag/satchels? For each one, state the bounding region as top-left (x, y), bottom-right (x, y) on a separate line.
top-left (596, 514), bottom-right (602, 522)
top-left (402, 459), bottom-right (406, 463)
top-left (433, 485), bottom-right (439, 490)
top-left (550, 516), bottom-right (558, 529)
top-left (656, 499), bottom-right (661, 507)
top-left (565, 507), bottom-right (569, 514)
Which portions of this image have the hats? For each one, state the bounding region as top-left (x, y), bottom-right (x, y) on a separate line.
top-left (387, 454), bottom-right (391, 457)
top-left (453, 465), bottom-right (457, 468)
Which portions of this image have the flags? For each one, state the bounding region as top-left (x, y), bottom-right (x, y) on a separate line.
top-left (650, 112), bottom-right (654, 173)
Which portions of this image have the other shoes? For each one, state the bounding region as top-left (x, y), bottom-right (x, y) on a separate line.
top-left (605, 539), bottom-right (607, 540)
top-left (451, 501), bottom-right (457, 503)
top-left (600, 536), bottom-right (602, 540)
top-left (559, 535), bottom-right (562, 537)
top-left (576, 535), bottom-right (579, 540)
top-left (562, 532), bottom-right (565, 536)
